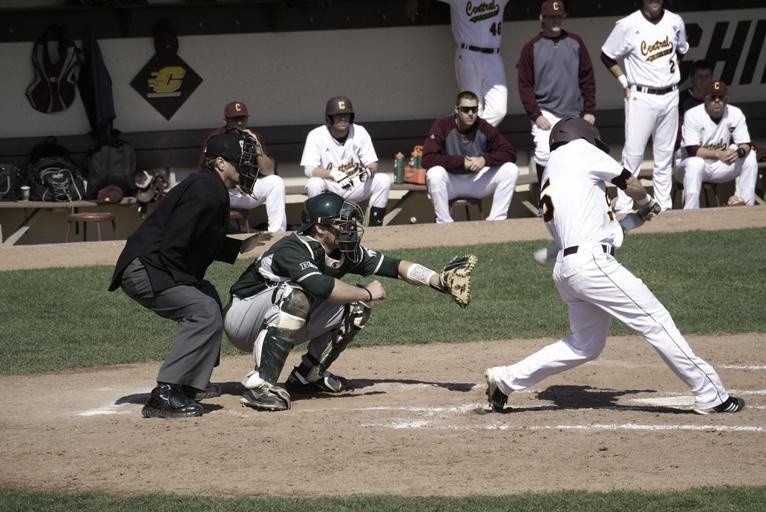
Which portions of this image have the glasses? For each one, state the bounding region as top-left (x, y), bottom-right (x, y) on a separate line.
top-left (457, 106), bottom-right (479, 114)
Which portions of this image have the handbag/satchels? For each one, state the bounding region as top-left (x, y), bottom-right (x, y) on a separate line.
top-left (0, 161), bottom-right (21, 200)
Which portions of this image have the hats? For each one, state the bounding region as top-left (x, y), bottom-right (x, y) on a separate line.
top-left (323, 95), bottom-right (356, 126)
top-left (540, 0), bottom-right (566, 16)
top-left (223, 101), bottom-right (250, 122)
top-left (202, 127), bottom-right (260, 166)
top-left (703, 80), bottom-right (730, 100)
top-left (96, 184), bottom-right (125, 205)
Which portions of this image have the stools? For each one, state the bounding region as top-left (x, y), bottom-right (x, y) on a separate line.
top-left (67, 212), bottom-right (118, 243)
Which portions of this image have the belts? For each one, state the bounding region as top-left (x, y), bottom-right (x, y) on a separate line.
top-left (562, 243), bottom-right (616, 258)
top-left (627, 82), bottom-right (681, 96)
top-left (460, 42), bottom-right (502, 55)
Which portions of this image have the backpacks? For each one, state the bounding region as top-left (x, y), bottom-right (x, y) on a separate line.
top-left (81, 130), bottom-right (140, 197)
top-left (24, 135), bottom-right (88, 201)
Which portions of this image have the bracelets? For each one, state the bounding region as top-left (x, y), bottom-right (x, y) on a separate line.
top-left (737, 148), bottom-right (746, 161)
top-left (617, 74), bottom-right (628, 90)
top-left (406, 264), bottom-right (436, 288)
top-left (361, 285), bottom-right (373, 303)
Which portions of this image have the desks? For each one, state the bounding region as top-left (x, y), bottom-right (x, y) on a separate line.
top-left (0, 197), bottom-right (153, 247)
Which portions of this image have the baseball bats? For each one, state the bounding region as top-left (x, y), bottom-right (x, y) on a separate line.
top-left (534, 203), bottom-right (660, 266)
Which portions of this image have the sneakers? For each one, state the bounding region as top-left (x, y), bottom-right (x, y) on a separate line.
top-left (187, 379), bottom-right (221, 402)
top-left (141, 383), bottom-right (205, 419)
top-left (691, 397), bottom-right (747, 415)
top-left (286, 366), bottom-right (352, 393)
top-left (484, 366), bottom-right (510, 414)
top-left (238, 373), bottom-right (293, 412)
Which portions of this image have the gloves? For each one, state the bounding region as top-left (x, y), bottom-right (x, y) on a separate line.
top-left (618, 212), bottom-right (645, 232)
top-left (637, 195), bottom-right (664, 221)
top-left (329, 167), bottom-right (356, 191)
top-left (357, 165), bottom-right (374, 184)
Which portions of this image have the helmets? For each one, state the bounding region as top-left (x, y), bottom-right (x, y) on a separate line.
top-left (297, 191), bottom-right (353, 231)
top-left (548, 115), bottom-right (612, 155)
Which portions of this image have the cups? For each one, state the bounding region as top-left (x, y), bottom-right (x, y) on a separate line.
top-left (21, 185), bottom-right (31, 201)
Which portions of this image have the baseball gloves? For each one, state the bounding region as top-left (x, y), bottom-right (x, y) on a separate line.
top-left (441, 254), bottom-right (479, 307)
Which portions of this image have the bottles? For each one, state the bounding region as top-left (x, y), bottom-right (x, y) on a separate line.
top-left (393, 150), bottom-right (405, 184)
top-left (408, 148), bottom-right (424, 171)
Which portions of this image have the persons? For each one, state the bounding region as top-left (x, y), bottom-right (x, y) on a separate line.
top-left (600, 0), bottom-right (690, 213)
top-left (420, 92), bottom-right (520, 222)
top-left (107, 136), bottom-right (274, 420)
top-left (299, 97), bottom-right (392, 227)
top-left (517, 2), bottom-right (596, 186)
top-left (222, 192), bottom-right (478, 412)
top-left (679, 60), bottom-right (714, 117)
top-left (199, 101), bottom-right (288, 232)
top-left (444, 0), bottom-right (511, 128)
top-left (484, 117), bottom-right (746, 414)
top-left (673, 82), bottom-right (759, 209)
